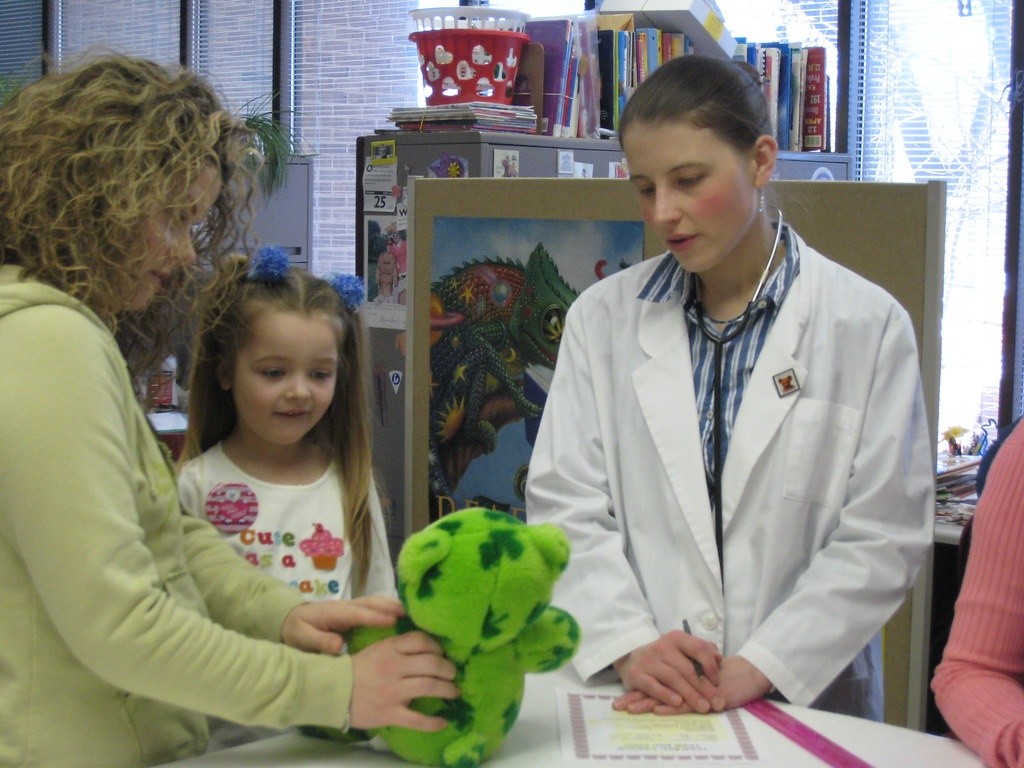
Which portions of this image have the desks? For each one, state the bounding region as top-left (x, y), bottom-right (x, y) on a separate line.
top-left (152, 667), bottom-right (988, 768)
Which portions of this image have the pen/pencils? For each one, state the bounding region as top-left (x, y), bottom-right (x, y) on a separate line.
top-left (683, 619), bottom-right (704, 680)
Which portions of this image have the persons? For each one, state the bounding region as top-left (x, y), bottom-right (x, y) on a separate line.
top-left (523, 56), bottom-right (935, 723)
top-left (2, 44), bottom-right (457, 768)
top-left (175, 248), bottom-right (402, 752)
top-left (930, 419), bottom-right (1024, 768)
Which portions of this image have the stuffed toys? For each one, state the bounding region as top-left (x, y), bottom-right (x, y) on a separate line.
top-left (300, 505), bottom-right (581, 768)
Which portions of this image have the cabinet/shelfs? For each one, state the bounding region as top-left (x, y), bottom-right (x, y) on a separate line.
top-left (195, 155), bottom-right (313, 272)
top-left (355, 131), bottom-right (855, 577)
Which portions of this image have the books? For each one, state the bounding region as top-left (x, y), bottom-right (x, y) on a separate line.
top-left (524, 12), bottom-right (826, 153)
